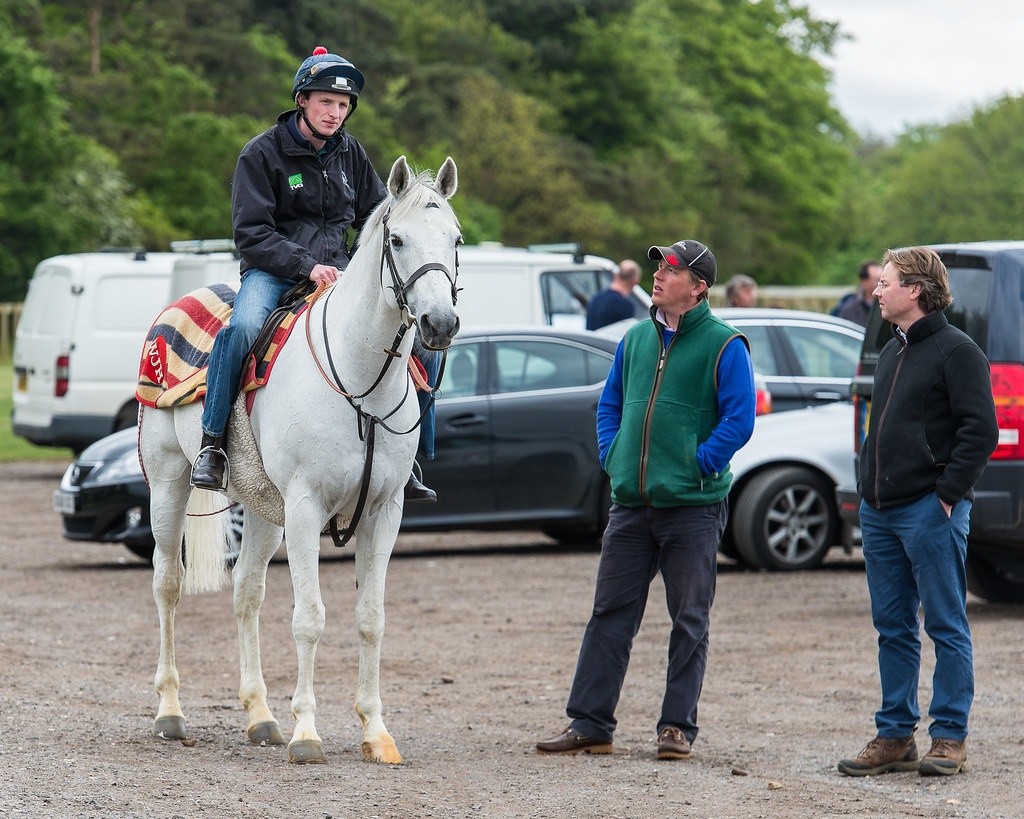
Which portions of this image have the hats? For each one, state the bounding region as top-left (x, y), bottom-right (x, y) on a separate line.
top-left (291, 47), bottom-right (365, 101)
top-left (648, 240), bottom-right (716, 288)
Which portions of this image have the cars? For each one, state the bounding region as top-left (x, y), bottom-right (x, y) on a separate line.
top-left (596, 308), bottom-right (866, 417)
top-left (52, 325), bottom-right (772, 560)
top-left (850, 241), bottom-right (1024, 605)
top-left (718, 400), bottom-right (861, 573)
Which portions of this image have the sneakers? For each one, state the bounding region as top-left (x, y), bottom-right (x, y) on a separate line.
top-left (918, 738), bottom-right (966, 775)
top-left (837, 727), bottom-right (919, 775)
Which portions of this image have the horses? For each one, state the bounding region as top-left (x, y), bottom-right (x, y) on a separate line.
top-left (136, 155), bottom-right (465, 765)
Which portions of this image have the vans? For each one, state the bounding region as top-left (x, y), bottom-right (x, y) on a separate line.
top-left (10, 238), bottom-right (236, 459)
top-left (170, 244), bottom-right (653, 394)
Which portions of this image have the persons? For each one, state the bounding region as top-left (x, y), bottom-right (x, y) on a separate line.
top-left (838, 246), bottom-right (999, 776)
top-left (828, 259), bottom-right (884, 329)
top-left (722, 274), bottom-right (756, 309)
top-left (586, 258), bottom-right (642, 332)
top-left (536, 239), bottom-right (757, 758)
top-left (191, 47), bottom-right (442, 504)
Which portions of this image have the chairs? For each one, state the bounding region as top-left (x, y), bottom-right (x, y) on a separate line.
top-left (451, 350), bottom-right (473, 399)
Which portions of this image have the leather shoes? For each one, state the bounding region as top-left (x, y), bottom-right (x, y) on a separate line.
top-left (192, 432), bottom-right (227, 486)
top-left (658, 727), bottom-right (690, 758)
top-left (404, 471), bottom-right (437, 503)
top-left (536, 727), bottom-right (614, 754)
